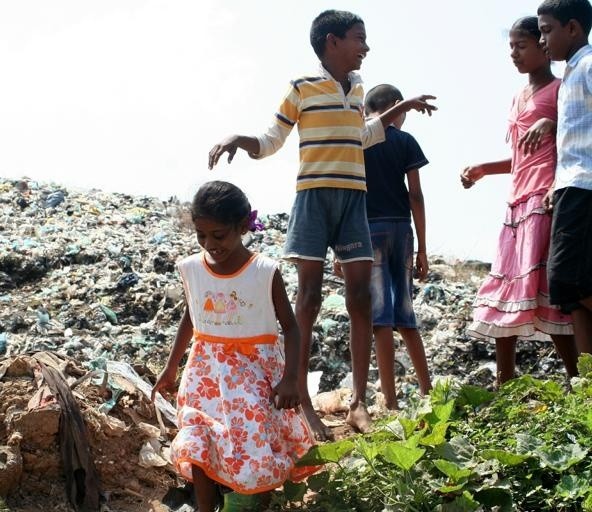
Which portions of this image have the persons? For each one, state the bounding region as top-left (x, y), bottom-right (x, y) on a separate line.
top-left (460, 15), bottom-right (582, 386)
top-left (538, 0), bottom-right (592, 357)
top-left (150, 178), bottom-right (301, 512)
top-left (332, 83), bottom-right (434, 408)
top-left (209, 6), bottom-right (440, 440)
